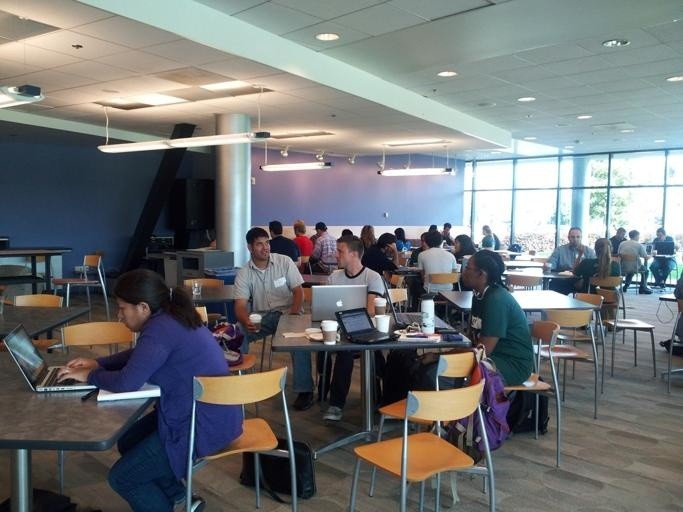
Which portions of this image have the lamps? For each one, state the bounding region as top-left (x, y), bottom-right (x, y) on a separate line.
top-left (377, 144), bottom-right (457, 176)
top-left (92, 88), bottom-right (273, 155)
top-left (254, 131), bottom-right (335, 173)
top-left (274, 139), bottom-right (415, 172)
top-left (0, 83), bottom-right (44, 110)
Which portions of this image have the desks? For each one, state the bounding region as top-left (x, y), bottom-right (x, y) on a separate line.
top-left (0, 301), bottom-right (91, 345)
top-left (271, 310), bottom-right (474, 459)
top-left (0, 361), bottom-right (161, 512)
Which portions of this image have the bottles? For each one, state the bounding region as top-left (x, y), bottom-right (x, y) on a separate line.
top-left (419, 295), bottom-right (436, 333)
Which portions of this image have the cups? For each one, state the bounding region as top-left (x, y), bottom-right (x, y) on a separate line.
top-left (320, 320), bottom-right (338, 345)
top-left (191, 279), bottom-right (201, 295)
top-left (248, 313), bottom-right (264, 332)
top-left (404, 252), bottom-right (411, 258)
top-left (374, 315), bottom-right (391, 334)
top-left (653, 250), bottom-right (657, 256)
top-left (544, 262), bottom-right (551, 273)
top-left (371, 296), bottom-right (387, 314)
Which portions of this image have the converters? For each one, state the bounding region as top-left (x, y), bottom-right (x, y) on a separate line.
top-left (436, 329), bottom-right (458, 334)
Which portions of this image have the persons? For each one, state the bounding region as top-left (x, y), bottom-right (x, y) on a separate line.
top-left (57, 267), bottom-right (244, 512)
top-left (414, 248), bottom-right (534, 391)
top-left (266, 219), bottom-right (501, 292)
top-left (232, 226), bottom-right (315, 411)
top-left (542, 226), bottom-right (683, 348)
top-left (316, 234), bottom-right (385, 422)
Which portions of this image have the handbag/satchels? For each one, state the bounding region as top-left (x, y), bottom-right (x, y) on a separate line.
top-left (240, 438), bottom-right (317, 499)
top-left (507, 377), bottom-right (550, 434)
top-left (208, 322), bottom-right (244, 366)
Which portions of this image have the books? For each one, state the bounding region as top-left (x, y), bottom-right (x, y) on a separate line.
top-left (96, 382), bottom-right (161, 401)
top-left (397, 332), bottom-right (440, 343)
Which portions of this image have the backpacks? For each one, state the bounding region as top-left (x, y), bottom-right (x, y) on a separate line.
top-left (450, 343), bottom-right (511, 453)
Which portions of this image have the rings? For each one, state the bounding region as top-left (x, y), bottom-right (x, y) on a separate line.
top-left (64, 368), bottom-right (69, 373)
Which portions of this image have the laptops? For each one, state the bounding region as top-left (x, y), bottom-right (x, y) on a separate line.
top-left (3, 322), bottom-right (101, 393)
top-left (311, 284), bottom-right (368, 322)
top-left (379, 274), bottom-right (422, 328)
top-left (334, 307), bottom-right (391, 344)
top-left (655, 241), bottom-right (677, 254)
top-left (640, 244), bottom-right (653, 257)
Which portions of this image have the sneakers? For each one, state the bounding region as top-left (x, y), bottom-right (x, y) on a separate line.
top-left (178, 494), bottom-right (205, 511)
top-left (322, 406), bottom-right (341, 421)
top-left (293, 393), bottom-right (312, 410)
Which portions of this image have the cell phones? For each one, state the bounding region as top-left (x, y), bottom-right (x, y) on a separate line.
top-left (444, 333), bottom-right (462, 341)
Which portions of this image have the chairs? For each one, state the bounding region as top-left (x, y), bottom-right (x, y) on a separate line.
top-left (0, 241), bottom-right (241, 310)
top-left (58, 320), bottom-right (140, 492)
top-left (366, 346), bottom-right (495, 501)
top-left (482, 320), bottom-right (565, 471)
top-left (184, 233), bottom-right (683, 392)
top-left (185, 365), bottom-right (301, 511)
top-left (529, 309), bottom-right (603, 421)
top-left (347, 378), bottom-right (499, 512)
top-left (13, 294), bottom-right (65, 356)
